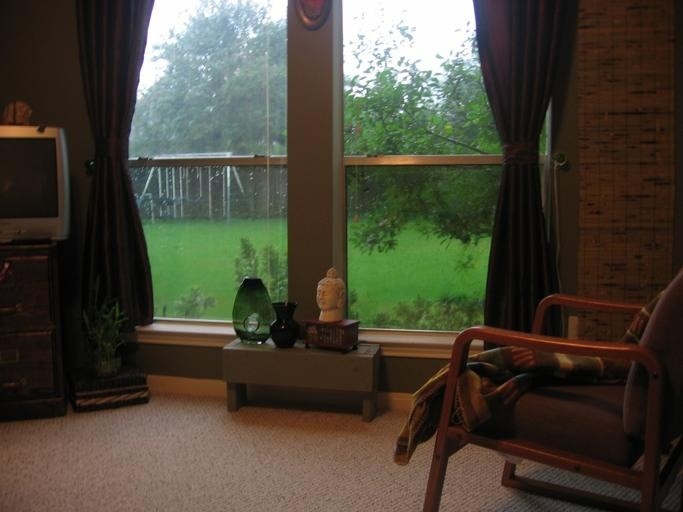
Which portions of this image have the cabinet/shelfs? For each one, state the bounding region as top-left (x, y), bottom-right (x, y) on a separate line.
top-left (0, 243), bottom-right (67, 422)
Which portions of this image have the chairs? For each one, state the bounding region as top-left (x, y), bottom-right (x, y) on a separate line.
top-left (393, 268), bottom-right (683, 512)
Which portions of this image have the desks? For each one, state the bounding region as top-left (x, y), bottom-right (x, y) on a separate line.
top-left (221, 337), bottom-right (381, 421)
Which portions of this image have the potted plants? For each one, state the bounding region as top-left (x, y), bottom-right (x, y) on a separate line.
top-left (71, 304), bottom-right (150, 413)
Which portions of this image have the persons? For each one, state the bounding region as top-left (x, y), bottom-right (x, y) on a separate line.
top-left (316, 267), bottom-right (347, 323)
top-left (506, 345), bottom-right (637, 381)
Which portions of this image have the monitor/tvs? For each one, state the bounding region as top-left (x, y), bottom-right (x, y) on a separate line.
top-left (0, 126), bottom-right (81, 246)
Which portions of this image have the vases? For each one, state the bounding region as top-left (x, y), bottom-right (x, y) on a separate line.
top-left (270, 302), bottom-right (300, 348)
